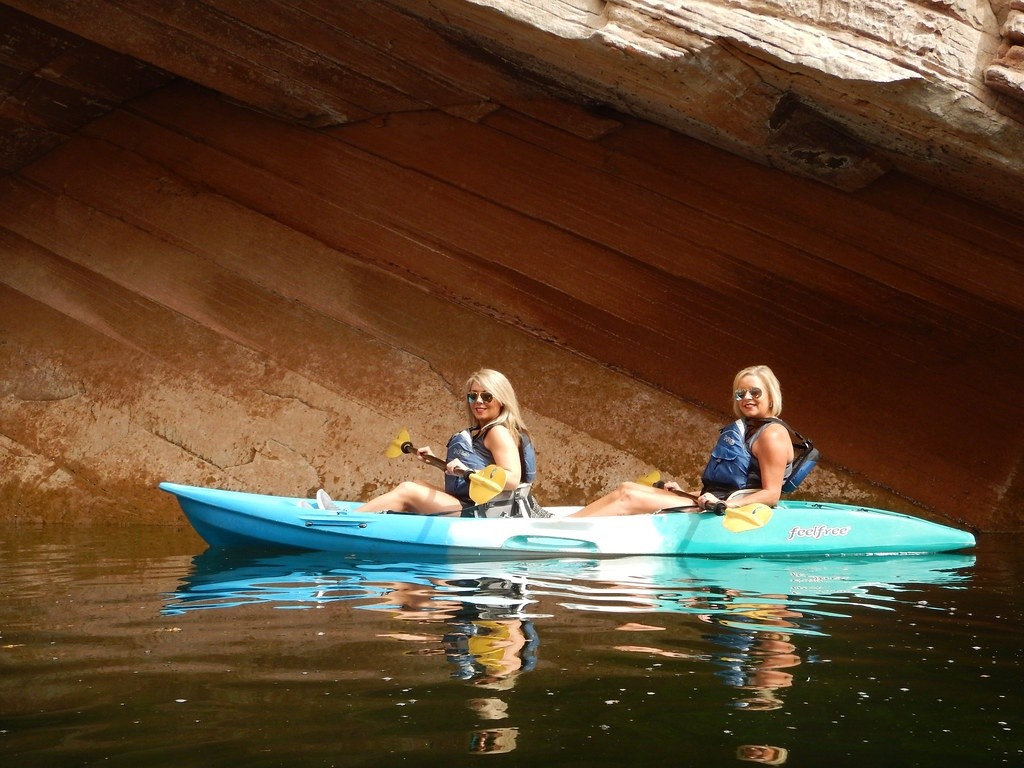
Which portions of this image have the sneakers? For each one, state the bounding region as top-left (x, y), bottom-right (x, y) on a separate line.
top-left (297, 499), bottom-right (315, 510)
top-left (317, 489), bottom-right (340, 510)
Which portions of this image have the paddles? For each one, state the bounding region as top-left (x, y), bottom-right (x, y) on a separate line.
top-left (635, 470), bottom-right (773, 532)
top-left (384, 428), bottom-right (509, 508)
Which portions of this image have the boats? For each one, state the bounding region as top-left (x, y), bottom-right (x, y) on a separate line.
top-left (160, 480), bottom-right (977, 555)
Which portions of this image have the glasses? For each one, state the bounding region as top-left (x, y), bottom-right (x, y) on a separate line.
top-left (734, 387), bottom-right (764, 400)
top-left (466, 392), bottom-right (496, 404)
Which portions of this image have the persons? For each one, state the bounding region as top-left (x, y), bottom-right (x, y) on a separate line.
top-left (569, 364), bottom-right (794, 518)
top-left (300, 370), bottom-right (537, 517)
